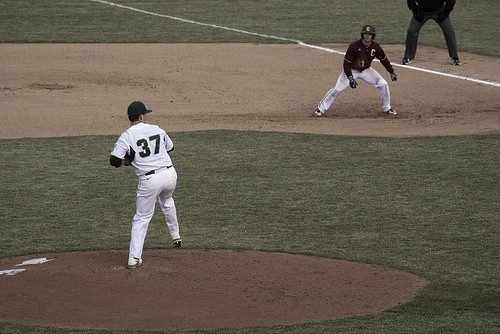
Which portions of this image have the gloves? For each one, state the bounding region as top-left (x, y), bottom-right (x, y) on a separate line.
top-left (349, 80), bottom-right (358, 89)
top-left (392, 73), bottom-right (398, 82)
top-left (436, 10), bottom-right (447, 22)
top-left (412, 10), bottom-right (425, 22)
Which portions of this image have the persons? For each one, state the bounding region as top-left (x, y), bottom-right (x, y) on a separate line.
top-left (109, 101), bottom-right (182, 268)
top-left (314, 25), bottom-right (398, 116)
top-left (400, 0), bottom-right (462, 65)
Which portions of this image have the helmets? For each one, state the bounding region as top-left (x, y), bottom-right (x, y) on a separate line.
top-left (361, 25), bottom-right (376, 41)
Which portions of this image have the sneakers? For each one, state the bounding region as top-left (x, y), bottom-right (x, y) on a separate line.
top-left (314, 107), bottom-right (323, 116)
top-left (127, 259), bottom-right (142, 268)
top-left (387, 108), bottom-right (397, 115)
top-left (173, 239), bottom-right (183, 246)
top-left (401, 58), bottom-right (413, 65)
top-left (451, 59), bottom-right (461, 65)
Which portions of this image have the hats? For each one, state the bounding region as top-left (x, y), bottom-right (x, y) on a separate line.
top-left (127, 101), bottom-right (153, 116)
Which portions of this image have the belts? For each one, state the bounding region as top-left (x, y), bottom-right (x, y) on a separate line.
top-left (145, 165), bottom-right (172, 176)
